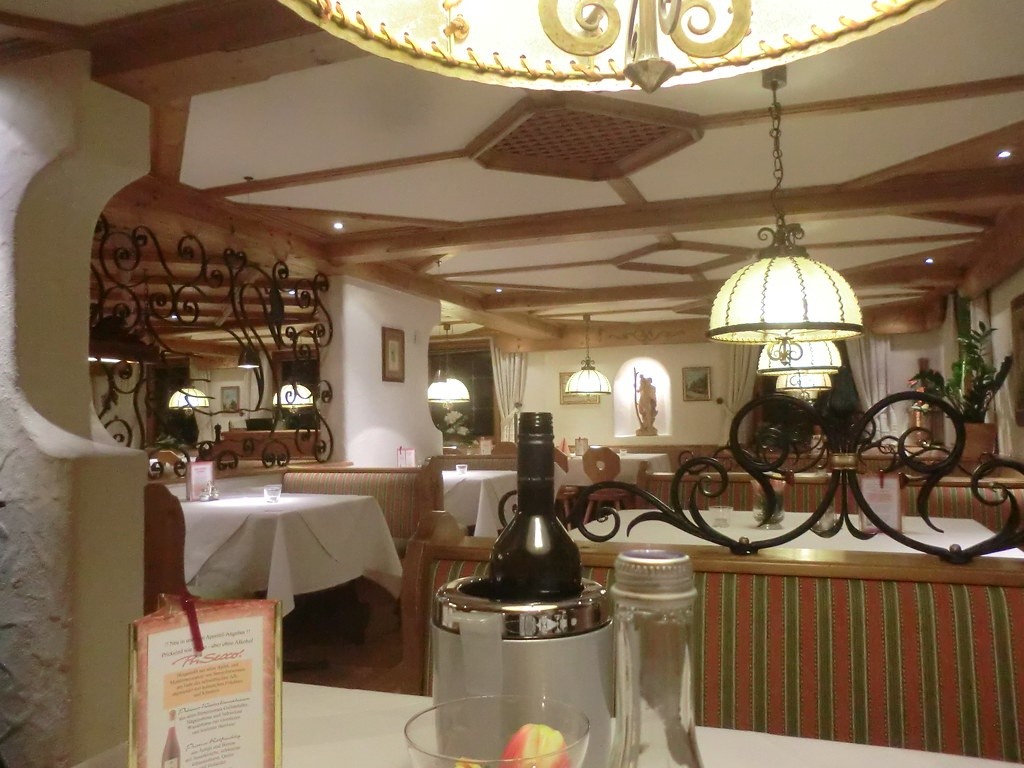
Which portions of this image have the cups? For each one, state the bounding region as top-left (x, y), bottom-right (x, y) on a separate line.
top-left (818, 495), bottom-right (835, 532)
top-left (456, 464), bottom-right (467, 475)
top-left (429, 574), bottom-right (615, 768)
top-left (404, 695), bottom-right (591, 768)
top-left (620, 449), bottom-right (627, 457)
top-left (263, 484), bottom-right (282, 503)
top-left (709, 506), bottom-right (733, 528)
top-left (749, 479), bottom-right (786, 530)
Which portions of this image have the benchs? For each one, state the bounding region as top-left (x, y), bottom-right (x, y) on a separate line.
top-left (117, 421), bottom-right (1024, 768)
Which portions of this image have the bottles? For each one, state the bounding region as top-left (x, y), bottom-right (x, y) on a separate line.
top-left (604, 549), bottom-right (706, 768)
top-left (490, 412), bottom-right (583, 601)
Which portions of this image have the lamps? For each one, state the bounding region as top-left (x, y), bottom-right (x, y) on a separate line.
top-left (274, 350), bottom-right (314, 413)
top-left (706, 65), bottom-right (862, 407)
top-left (564, 314), bottom-right (611, 396)
top-left (428, 323), bottom-right (470, 406)
top-left (168, 354), bottom-right (210, 416)
top-left (237, 341), bottom-right (260, 370)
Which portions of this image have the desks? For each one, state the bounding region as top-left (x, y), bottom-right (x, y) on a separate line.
top-left (279, 681), bottom-right (1024, 768)
top-left (565, 507), bottom-right (1024, 569)
top-left (177, 493), bottom-right (404, 618)
top-left (442, 470), bottom-right (519, 539)
top-left (553, 451), bottom-right (671, 487)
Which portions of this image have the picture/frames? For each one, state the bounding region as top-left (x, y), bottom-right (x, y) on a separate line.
top-left (220, 386), bottom-right (240, 412)
top-left (682, 367), bottom-right (712, 402)
top-left (382, 327), bottom-right (405, 383)
top-left (559, 372), bottom-right (600, 405)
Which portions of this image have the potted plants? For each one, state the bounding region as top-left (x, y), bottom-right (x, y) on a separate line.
top-left (911, 319), bottom-right (1012, 460)
